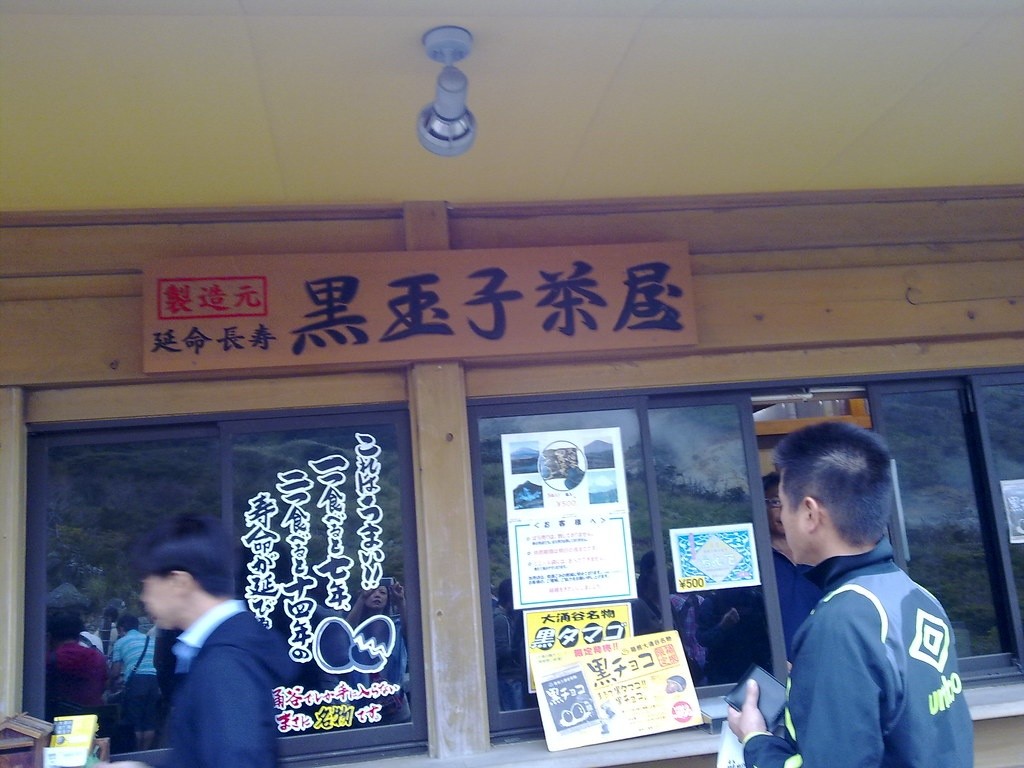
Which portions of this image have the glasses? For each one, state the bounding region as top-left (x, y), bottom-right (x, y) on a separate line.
top-left (767, 498), bottom-right (781, 510)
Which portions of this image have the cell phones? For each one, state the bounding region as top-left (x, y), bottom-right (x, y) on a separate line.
top-left (379, 578), bottom-right (394, 586)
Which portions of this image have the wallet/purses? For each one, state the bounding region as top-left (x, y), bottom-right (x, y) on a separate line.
top-left (724, 669), bottom-right (789, 730)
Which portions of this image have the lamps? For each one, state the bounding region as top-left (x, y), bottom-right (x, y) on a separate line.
top-left (416, 65), bottom-right (478, 157)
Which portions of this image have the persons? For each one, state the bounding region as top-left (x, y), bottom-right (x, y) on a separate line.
top-left (307, 581), bottom-right (410, 722)
top-left (493, 549), bottom-right (756, 718)
top-left (727, 422), bottom-right (974, 768)
top-left (763, 469), bottom-right (828, 672)
top-left (111, 514), bottom-right (292, 768)
top-left (47, 605), bottom-right (159, 752)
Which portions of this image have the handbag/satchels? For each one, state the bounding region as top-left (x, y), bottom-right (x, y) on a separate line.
top-left (716, 721), bottom-right (746, 768)
top-left (108, 681), bottom-right (143, 721)
